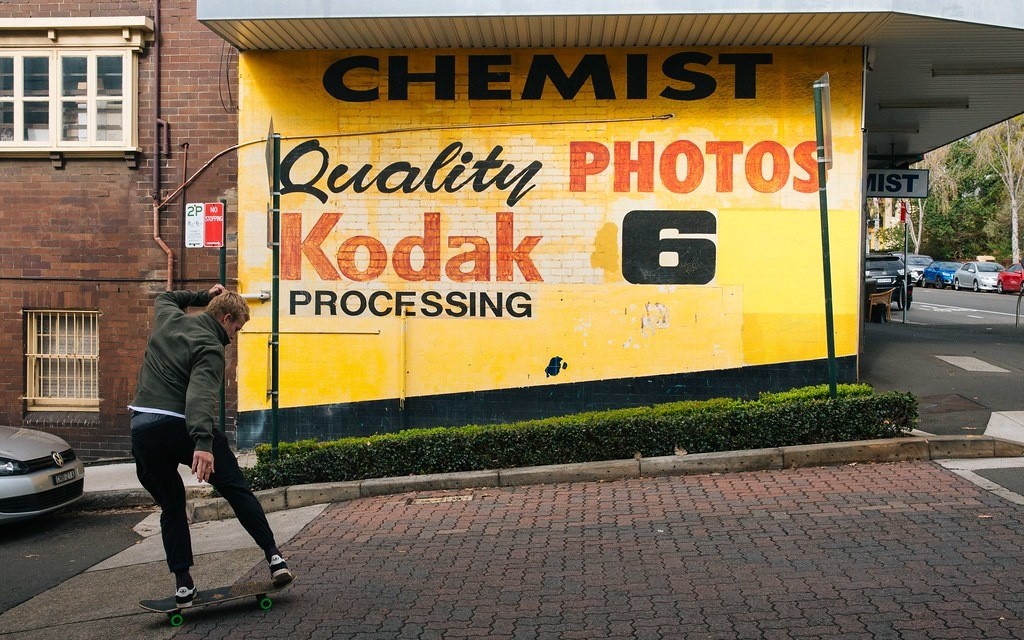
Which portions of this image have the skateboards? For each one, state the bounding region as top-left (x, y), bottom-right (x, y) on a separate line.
top-left (137, 574), bottom-right (299, 627)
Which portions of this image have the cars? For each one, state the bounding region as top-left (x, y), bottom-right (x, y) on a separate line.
top-left (997, 263), bottom-right (1024, 295)
top-left (922, 261), bottom-right (964, 289)
top-left (953, 261), bottom-right (1005, 292)
top-left (0, 426), bottom-right (85, 531)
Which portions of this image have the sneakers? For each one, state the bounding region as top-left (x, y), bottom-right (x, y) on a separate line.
top-left (175, 586), bottom-right (197, 608)
top-left (268, 554), bottom-right (292, 583)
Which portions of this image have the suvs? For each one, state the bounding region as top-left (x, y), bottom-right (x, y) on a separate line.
top-left (894, 254), bottom-right (934, 286)
top-left (864, 252), bottom-right (913, 313)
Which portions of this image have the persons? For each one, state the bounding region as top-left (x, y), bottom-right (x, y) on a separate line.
top-left (128, 283), bottom-right (294, 609)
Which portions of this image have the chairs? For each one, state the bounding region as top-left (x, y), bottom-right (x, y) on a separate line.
top-left (869, 287), bottom-right (896, 323)
